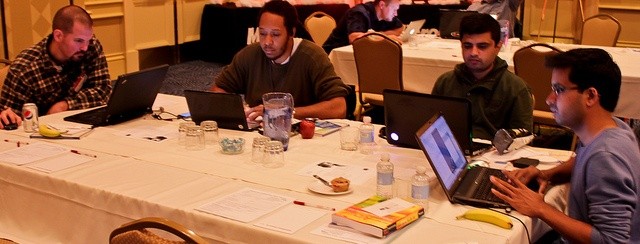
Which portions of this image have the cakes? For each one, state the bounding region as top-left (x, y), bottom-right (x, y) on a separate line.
top-left (332, 175), bottom-right (351, 194)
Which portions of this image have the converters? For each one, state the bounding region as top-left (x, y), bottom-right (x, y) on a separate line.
top-left (508, 157), bottom-right (539, 168)
top-left (152, 107), bottom-right (179, 120)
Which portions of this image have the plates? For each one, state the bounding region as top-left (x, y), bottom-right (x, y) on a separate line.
top-left (307, 172), bottom-right (355, 195)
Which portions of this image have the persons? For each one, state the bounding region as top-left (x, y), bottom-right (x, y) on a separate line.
top-left (466, 0), bottom-right (514, 38)
top-left (0, 5), bottom-right (113, 129)
top-left (321, 0), bottom-right (405, 55)
top-left (490, 48), bottom-right (640, 244)
top-left (208, 0), bottom-right (351, 121)
top-left (431, 13), bottom-right (533, 147)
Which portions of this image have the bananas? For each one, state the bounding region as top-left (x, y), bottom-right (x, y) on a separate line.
top-left (456, 207), bottom-right (513, 229)
top-left (37, 121), bottom-right (70, 138)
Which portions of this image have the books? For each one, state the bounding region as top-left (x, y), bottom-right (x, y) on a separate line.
top-left (328, 194), bottom-right (424, 240)
top-left (314, 120), bottom-right (350, 137)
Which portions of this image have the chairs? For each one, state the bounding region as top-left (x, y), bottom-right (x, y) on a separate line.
top-left (580, 13), bottom-right (621, 47)
top-left (303, 12), bottom-right (336, 48)
top-left (513, 43), bottom-right (566, 148)
top-left (108, 216), bottom-right (209, 244)
top-left (352, 32), bottom-right (404, 123)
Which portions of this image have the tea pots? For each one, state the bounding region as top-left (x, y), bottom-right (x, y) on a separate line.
top-left (262, 91), bottom-right (295, 152)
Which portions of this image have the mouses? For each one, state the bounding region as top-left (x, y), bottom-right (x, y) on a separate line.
top-left (4, 120), bottom-right (18, 130)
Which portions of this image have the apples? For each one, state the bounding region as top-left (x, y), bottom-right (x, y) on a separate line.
top-left (299, 121), bottom-right (316, 139)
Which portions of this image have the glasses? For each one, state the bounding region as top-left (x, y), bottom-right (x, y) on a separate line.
top-left (551, 81), bottom-right (577, 94)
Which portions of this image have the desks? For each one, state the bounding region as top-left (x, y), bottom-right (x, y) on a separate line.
top-left (0, 92), bottom-right (576, 244)
top-left (329, 32), bottom-right (640, 121)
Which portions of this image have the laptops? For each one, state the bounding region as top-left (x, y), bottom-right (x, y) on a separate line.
top-left (439, 10), bottom-right (479, 39)
top-left (395, 19), bottom-right (427, 42)
top-left (382, 88), bottom-right (493, 156)
top-left (184, 88), bottom-right (263, 132)
top-left (414, 109), bottom-right (536, 208)
top-left (63, 63), bottom-right (170, 129)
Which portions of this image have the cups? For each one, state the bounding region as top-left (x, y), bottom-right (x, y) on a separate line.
top-left (178, 118), bottom-right (220, 153)
top-left (339, 126), bottom-right (361, 151)
top-left (251, 134), bottom-right (283, 171)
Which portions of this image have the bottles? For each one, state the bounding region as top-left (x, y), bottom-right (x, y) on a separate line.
top-left (359, 116), bottom-right (378, 157)
top-left (376, 154), bottom-right (397, 198)
top-left (22, 102), bottom-right (38, 135)
top-left (409, 165), bottom-right (432, 214)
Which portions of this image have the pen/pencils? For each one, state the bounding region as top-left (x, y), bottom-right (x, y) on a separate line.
top-left (289, 199), bottom-right (338, 213)
top-left (4, 138), bottom-right (29, 145)
top-left (70, 149), bottom-right (97, 159)
